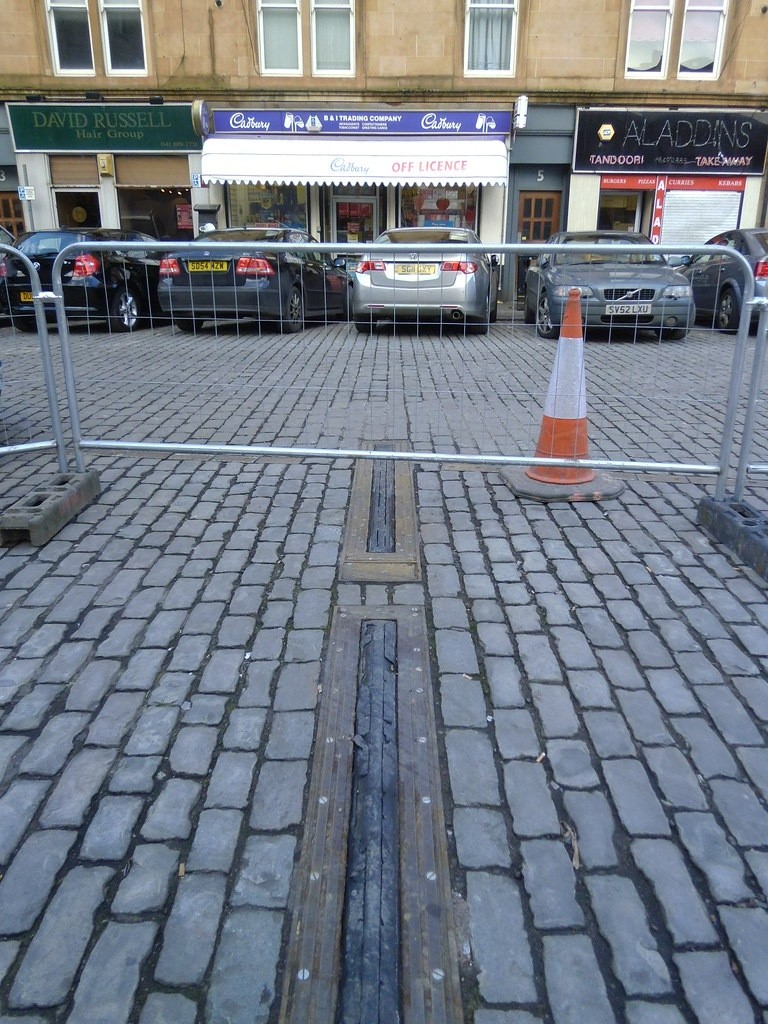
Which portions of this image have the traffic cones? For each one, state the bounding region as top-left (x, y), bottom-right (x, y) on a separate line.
top-left (496, 288), bottom-right (627, 503)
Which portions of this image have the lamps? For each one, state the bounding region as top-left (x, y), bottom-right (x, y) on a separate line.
top-left (26, 94), bottom-right (46, 102)
top-left (149, 96), bottom-right (164, 104)
top-left (85, 92), bottom-right (105, 100)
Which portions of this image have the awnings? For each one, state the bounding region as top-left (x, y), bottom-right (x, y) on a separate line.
top-left (201, 138), bottom-right (508, 188)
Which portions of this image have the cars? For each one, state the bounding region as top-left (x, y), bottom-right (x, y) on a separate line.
top-left (157, 228), bottom-right (352, 334)
top-left (677, 228), bottom-right (768, 335)
top-left (524, 230), bottom-right (696, 340)
top-left (0, 225), bottom-right (174, 332)
top-left (352, 227), bottom-right (499, 334)
